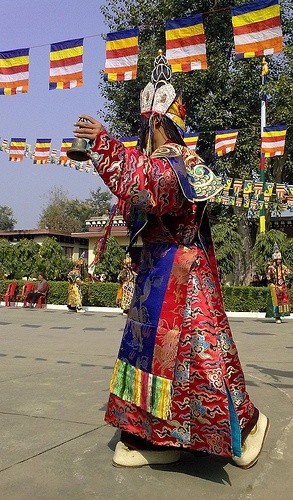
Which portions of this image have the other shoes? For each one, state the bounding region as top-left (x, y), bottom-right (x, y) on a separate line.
top-left (274, 319), bottom-right (282, 323)
top-left (111, 441), bottom-right (183, 469)
top-left (68, 308), bottom-right (76, 312)
top-left (76, 308), bottom-right (85, 313)
top-left (232, 406), bottom-right (270, 470)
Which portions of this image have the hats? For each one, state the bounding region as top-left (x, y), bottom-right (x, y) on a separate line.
top-left (38, 273), bottom-right (46, 279)
top-left (76, 251), bottom-right (86, 277)
top-left (139, 48), bottom-right (185, 157)
top-left (271, 241), bottom-right (285, 285)
top-left (123, 246), bottom-right (134, 285)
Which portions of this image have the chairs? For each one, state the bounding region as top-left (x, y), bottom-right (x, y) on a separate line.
top-left (16, 283), bottom-right (48, 309)
top-left (0, 281), bottom-right (18, 307)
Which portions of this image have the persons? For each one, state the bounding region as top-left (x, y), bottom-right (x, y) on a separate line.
top-left (67, 266), bottom-right (85, 313)
top-left (73, 49), bottom-right (270, 470)
top-left (25, 272), bottom-right (48, 308)
top-left (116, 257), bottom-right (138, 313)
top-left (266, 253), bottom-right (291, 323)
top-left (102, 208), bottom-right (110, 217)
